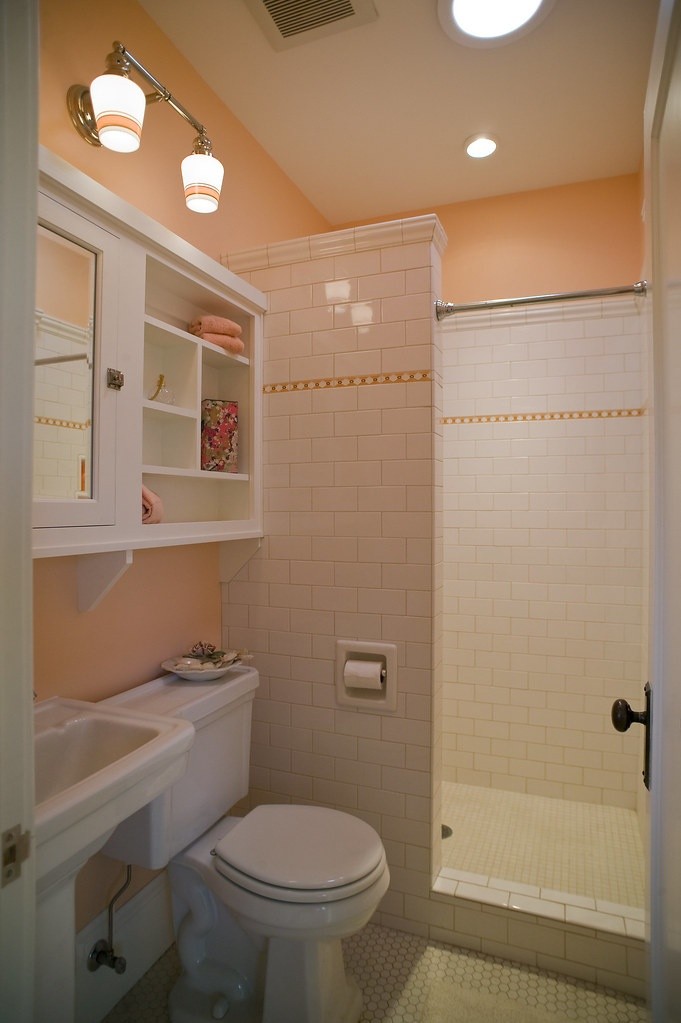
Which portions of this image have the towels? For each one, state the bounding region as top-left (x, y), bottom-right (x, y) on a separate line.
top-left (187, 316), bottom-right (245, 355)
top-left (143, 484), bottom-right (167, 525)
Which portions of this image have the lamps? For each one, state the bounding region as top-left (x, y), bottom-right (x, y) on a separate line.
top-left (66, 41), bottom-right (224, 214)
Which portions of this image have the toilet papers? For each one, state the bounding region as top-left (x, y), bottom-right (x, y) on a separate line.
top-left (343, 659), bottom-right (383, 690)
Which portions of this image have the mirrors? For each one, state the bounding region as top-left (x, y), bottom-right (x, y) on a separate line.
top-left (32, 215), bottom-right (97, 500)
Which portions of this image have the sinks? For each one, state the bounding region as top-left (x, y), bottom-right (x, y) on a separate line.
top-left (35, 697), bottom-right (196, 907)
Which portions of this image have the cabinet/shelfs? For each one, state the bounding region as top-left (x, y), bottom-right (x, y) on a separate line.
top-left (32, 144), bottom-right (267, 557)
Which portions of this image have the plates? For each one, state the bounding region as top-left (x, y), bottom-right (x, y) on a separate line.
top-left (159, 655), bottom-right (243, 682)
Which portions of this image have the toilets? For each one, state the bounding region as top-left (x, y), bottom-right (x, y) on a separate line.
top-left (93, 663), bottom-right (392, 1023)
